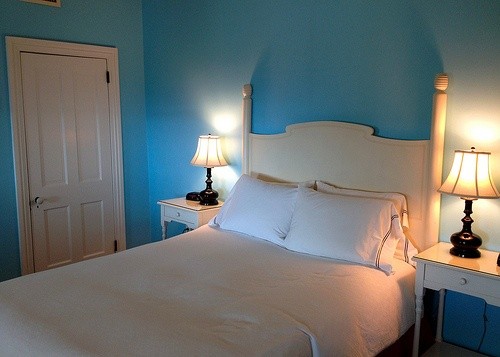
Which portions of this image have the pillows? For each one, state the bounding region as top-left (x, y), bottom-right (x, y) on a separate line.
top-left (211, 174), bottom-right (416, 275)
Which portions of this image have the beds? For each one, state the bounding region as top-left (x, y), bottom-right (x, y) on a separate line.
top-left (0, 73), bottom-right (449, 357)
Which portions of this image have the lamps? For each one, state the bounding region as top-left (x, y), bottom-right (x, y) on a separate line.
top-left (189, 132), bottom-right (228, 206)
top-left (438, 147), bottom-right (500, 259)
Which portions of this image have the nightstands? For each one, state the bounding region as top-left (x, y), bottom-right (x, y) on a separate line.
top-left (157, 197), bottom-right (224, 241)
top-left (411, 241), bottom-right (500, 357)
